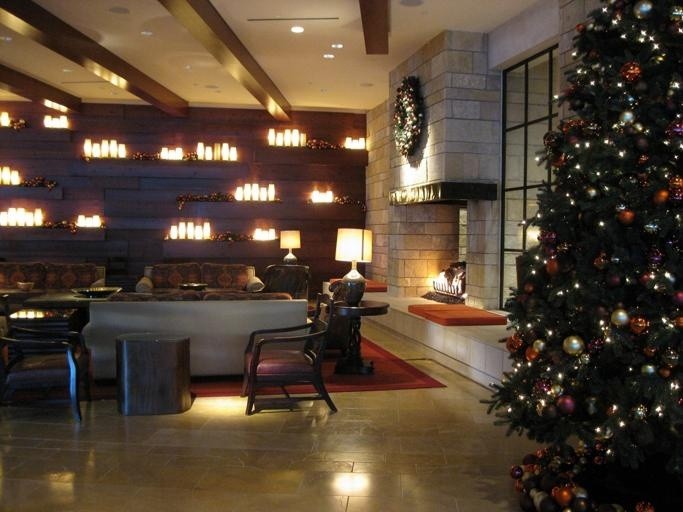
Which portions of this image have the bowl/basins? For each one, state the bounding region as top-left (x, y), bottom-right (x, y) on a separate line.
top-left (18, 281), bottom-right (34, 291)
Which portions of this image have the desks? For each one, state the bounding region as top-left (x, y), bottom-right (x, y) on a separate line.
top-left (7, 305), bottom-right (79, 361)
top-left (114, 331), bottom-right (192, 416)
top-left (0, 289), bottom-right (47, 312)
top-left (332, 300), bottom-right (389, 377)
top-left (21, 286), bottom-right (123, 308)
top-left (179, 281), bottom-right (207, 291)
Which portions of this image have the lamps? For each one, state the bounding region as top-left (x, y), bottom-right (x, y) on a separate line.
top-left (330, 226), bottom-right (372, 306)
top-left (279, 230), bottom-right (301, 265)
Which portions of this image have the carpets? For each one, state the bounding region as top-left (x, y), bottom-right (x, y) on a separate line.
top-left (0, 304), bottom-right (448, 398)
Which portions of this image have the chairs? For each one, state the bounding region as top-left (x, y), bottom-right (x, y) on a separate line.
top-left (0, 309), bottom-right (93, 422)
top-left (241, 292), bottom-right (341, 415)
top-left (260, 260), bottom-right (310, 302)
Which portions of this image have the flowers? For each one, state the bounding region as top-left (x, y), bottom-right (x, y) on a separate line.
top-left (173, 189), bottom-right (234, 212)
top-left (182, 151), bottom-right (199, 162)
top-left (127, 149), bottom-right (160, 161)
top-left (306, 138), bottom-right (348, 153)
top-left (210, 229), bottom-right (252, 249)
top-left (16, 173), bottom-right (58, 190)
top-left (6, 117), bottom-right (26, 132)
top-left (334, 194), bottom-right (366, 213)
top-left (39, 219), bottom-right (77, 235)
top-left (389, 73), bottom-right (425, 156)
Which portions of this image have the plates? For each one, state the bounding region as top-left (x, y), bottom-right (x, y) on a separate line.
top-left (70, 286), bottom-right (122, 296)
top-left (178, 283), bottom-right (209, 291)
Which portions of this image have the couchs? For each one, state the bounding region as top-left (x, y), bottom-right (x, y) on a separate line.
top-left (135, 261), bottom-right (266, 294)
top-left (0, 260), bottom-right (108, 297)
top-left (81, 290), bottom-right (314, 380)
